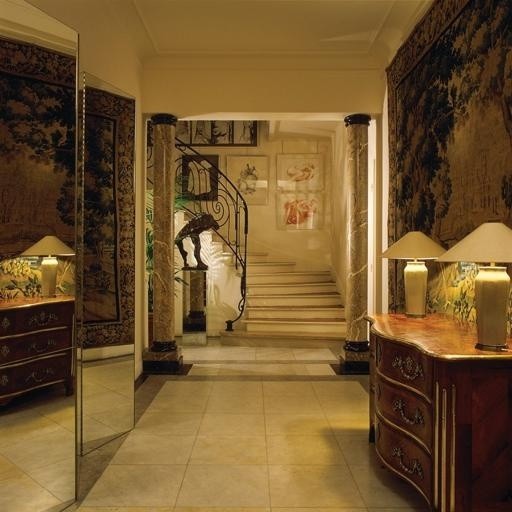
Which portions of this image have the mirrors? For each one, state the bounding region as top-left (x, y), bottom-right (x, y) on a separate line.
top-left (77, 66), bottom-right (138, 460)
top-left (0, 2), bottom-right (83, 512)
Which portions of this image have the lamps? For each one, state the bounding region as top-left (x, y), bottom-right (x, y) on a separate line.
top-left (436, 220), bottom-right (511, 354)
top-left (19, 232), bottom-right (76, 300)
top-left (381, 229), bottom-right (453, 317)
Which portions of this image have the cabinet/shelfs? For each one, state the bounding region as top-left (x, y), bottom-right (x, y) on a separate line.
top-left (0, 295), bottom-right (73, 416)
top-left (359, 310), bottom-right (511, 512)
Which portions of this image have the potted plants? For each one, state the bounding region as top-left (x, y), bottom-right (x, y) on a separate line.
top-left (145, 173), bottom-right (193, 347)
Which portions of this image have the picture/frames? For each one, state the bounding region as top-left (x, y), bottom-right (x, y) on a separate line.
top-left (225, 154), bottom-right (269, 207)
top-left (274, 191), bottom-right (325, 231)
top-left (147, 120), bottom-right (259, 148)
top-left (275, 152), bottom-right (326, 192)
top-left (181, 154), bottom-right (218, 202)
